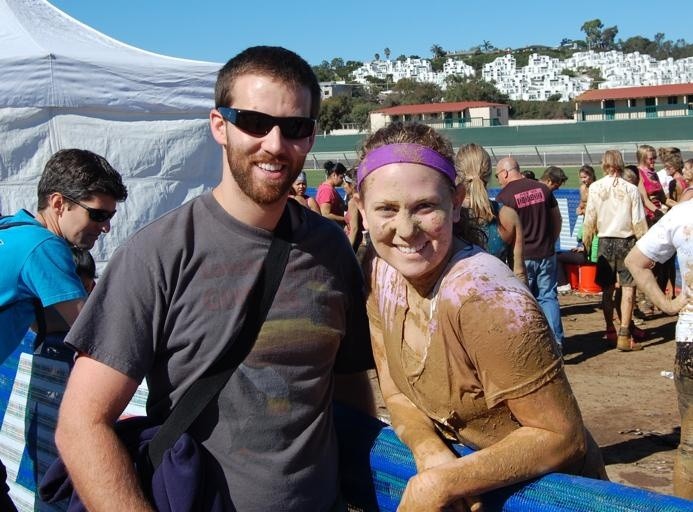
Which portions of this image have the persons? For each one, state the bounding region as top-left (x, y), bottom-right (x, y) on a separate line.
top-left (454, 144), bottom-right (568, 359)
top-left (288, 160), bottom-right (370, 256)
top-left (30, 245), bottom-right (99, 341)
top-left (0, 148), bottom-right (128, 512)
top-left (52, 44), bottom-right (381, 512)
top-left (573, 144), bottom-right (693, 351)
top-left (339, 121), bottom-right (614, 512)
top-left (622, 197), bottom-right (693, 505)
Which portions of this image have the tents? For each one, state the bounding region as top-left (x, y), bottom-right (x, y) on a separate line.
top-left (0, 0), bottom-right (232, 417)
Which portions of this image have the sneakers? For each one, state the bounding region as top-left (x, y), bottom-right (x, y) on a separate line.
top-left (607, 324), bottom-right (644, 352)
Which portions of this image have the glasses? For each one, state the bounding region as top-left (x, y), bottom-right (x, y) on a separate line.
top-left (217, 107), bottom-right (315, 140)
top-left (64, 195), bottom-right (117, 222)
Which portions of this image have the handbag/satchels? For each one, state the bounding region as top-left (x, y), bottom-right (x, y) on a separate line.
top-left (558, 248), bottom-right (587, 264)
